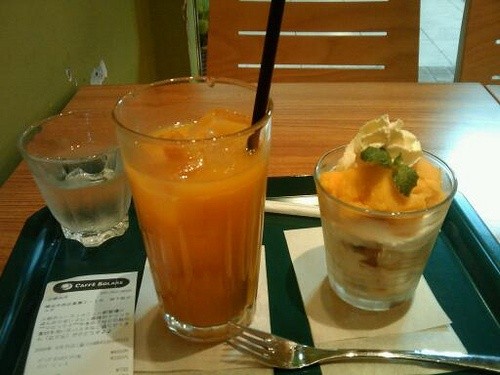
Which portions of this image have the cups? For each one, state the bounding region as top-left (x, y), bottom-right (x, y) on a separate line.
top-left (313, 145), bottom-right (459, 312)
top-left (18, 109), bottom-right (133, 247)
top-left (111, 76), bottom-right (274, 344)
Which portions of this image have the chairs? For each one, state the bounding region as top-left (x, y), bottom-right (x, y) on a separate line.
top-left (205, 0), bottom-right (420, 84)
top-left (454, 0), bottom-right (500, 82)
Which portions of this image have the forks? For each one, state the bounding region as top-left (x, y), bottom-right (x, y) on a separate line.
top-left (223, 320), bottom-right (500, 372)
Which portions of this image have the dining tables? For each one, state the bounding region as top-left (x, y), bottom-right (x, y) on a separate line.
top-left (0, 82), bottom-right (500, 275)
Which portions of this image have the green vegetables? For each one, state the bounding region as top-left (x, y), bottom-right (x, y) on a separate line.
top-left (360, 146), bottom-right (419, 197)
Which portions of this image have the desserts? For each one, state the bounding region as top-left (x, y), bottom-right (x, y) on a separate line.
top-left (316, 112), bottom-right (449, 311)
